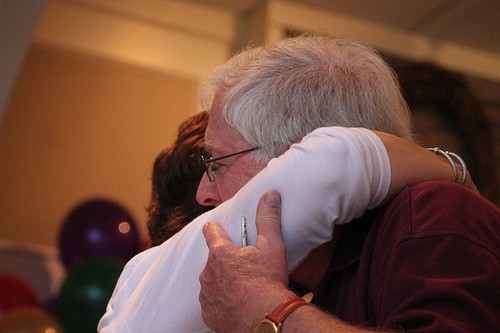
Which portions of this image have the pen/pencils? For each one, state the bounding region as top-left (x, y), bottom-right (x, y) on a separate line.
top-left (241, 216), bottom-right (247, 247)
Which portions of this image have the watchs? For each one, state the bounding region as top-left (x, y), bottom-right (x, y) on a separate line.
top-left (251, 297), bottom-right (310, 332)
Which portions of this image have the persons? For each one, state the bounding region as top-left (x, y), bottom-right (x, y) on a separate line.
top-left (196, 31), bottom-right (500, 333)
top-left (97, 108), bottom-right (480, 332)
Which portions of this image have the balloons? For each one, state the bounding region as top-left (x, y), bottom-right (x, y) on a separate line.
top-left (49, 266), bottom-right (121, 333)
top-left (56, 199), bottom-right (139, 273)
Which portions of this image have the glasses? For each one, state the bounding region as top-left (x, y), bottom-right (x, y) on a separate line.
top-left (200, 146), bottom-right (260, 183)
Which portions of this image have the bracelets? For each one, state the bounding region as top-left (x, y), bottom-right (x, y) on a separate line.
top-left (426, 146), bottom-right (467, 185)
